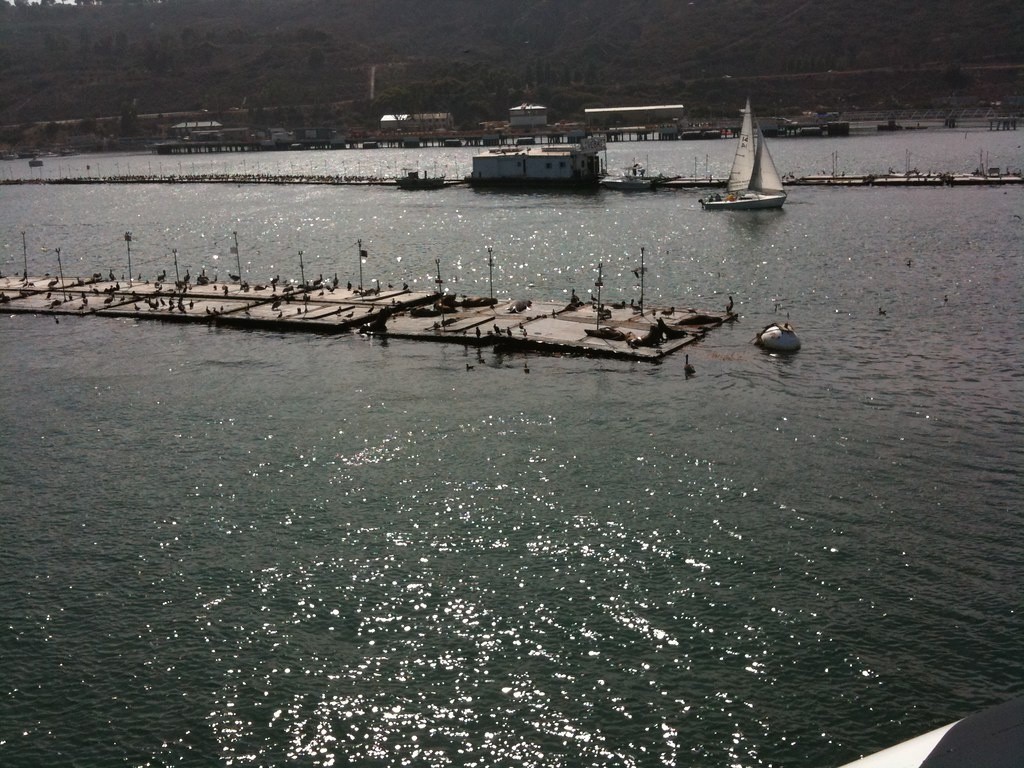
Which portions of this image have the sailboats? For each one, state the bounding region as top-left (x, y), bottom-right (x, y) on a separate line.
top-left (694, 98), bottom-right (788, 213)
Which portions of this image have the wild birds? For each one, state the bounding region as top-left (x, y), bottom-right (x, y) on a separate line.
top-left (0, 260), bottom-right (950, 378)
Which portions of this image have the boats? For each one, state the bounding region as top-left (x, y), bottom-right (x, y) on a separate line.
top-left (393, 171), bottom-right (445, 190)
top-left (600, 174), bottom-right (653, 193)
